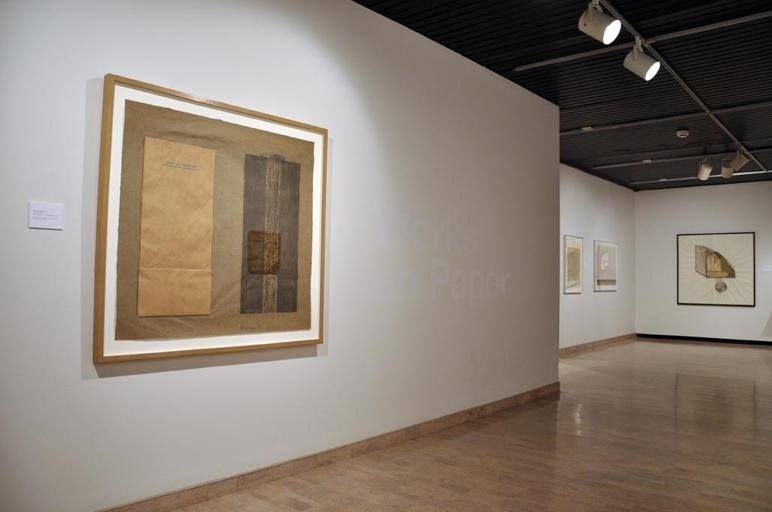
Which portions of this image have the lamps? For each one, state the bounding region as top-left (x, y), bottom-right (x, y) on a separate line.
top-left (576, 0), bottom-right (749, 182)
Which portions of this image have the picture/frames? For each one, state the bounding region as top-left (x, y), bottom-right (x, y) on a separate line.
top-left (564, 234), bottom-right (584, 294)
top-left (94, 73), bottom-right (327, 364)
top-left (677, 232), bottom-right (755, 307)
top-left (594, 240), bottom-right (618, 292)
